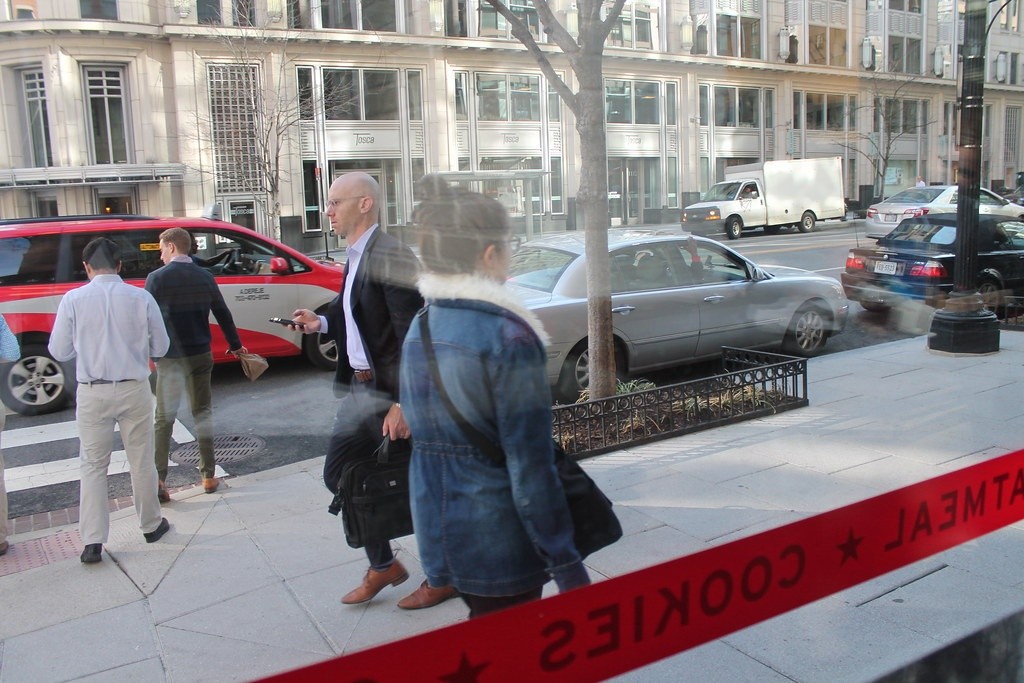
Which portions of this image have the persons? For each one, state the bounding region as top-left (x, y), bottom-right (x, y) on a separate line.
top-left (283, 170), bottom-right (462, 606)
top-left (0, 311), bottom-right (22, 556)
top-left (916, 175), bottom-right (925, 187)
top-left (145, 227), bottom-right (248, 502)
top-left (48, 238), bottom-right (172, 558)
top-left (741, 186), bottom-right (753, 198)
top-left (397, 173), bottom-right (623, 622)
top-left (189, 231), bottom-right (236, 267)
top-left (655, 236), bottom-right (702, 280)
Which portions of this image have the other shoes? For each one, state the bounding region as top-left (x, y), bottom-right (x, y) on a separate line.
top-left (203, 477), bottom-right (219, 492)
top-left (157, 484), bottom-right (169, 501)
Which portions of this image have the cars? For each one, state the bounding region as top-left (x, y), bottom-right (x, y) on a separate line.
top-left (1002, 184), bottom-right (1024, 206)
top-left (501, 228), bottom-right (850, 411)
top-left (839, 210), bottom-right (1024, 319)
top-left (864, 184), bottom-right (1024, 240)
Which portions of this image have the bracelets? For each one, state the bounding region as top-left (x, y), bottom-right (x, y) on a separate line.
top-left (392, 402), bottom-right (400, 407)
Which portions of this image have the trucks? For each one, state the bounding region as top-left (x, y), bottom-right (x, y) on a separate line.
top-left (678, 156), bottom-right (846, 240)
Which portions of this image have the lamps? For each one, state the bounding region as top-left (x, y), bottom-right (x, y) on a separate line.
top-left (266, 0), bottom-right (283, 23)
top-left (930, 46), bottom-right (944, 78)
top-left (777, 26), bottom-right (790, 60)
top-left (173, 0), bottom-right (191, 18)
top-left (994, 53), bottom-right (1007, 84)
top-left (678, 15), bottom-right (693, 52)
top-left (859, 37), bottom-right (872, 69)
top-left (563, 4), bottom-right (579, 41)
top-left (427, 0), bottom-right (444, 32)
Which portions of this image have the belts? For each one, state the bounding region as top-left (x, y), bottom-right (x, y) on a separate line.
top-left (82, 379), bottom-right (131, 384)
top-left (353, 370), bottom-right (372, 382)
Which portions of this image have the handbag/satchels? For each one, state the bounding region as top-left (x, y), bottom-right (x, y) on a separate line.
top-left (328, 435), bottom-right (414, 549)
top-left (537, 439), bottom-right (621, 586)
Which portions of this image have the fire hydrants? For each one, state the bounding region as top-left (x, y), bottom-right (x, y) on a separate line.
top-left (840, 203), bottom-right (848, 221)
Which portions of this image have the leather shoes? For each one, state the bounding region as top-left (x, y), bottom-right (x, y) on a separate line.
top-left (398, 578), bottom-right (457, 609)
top-left (143, 518), bottom-right (169, 543)
top-left (341, 558), bottom-right (410, 604)
top-left (80, 543), bottom-right (102, 562)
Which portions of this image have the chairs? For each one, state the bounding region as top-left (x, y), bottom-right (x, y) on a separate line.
top-left (630, 252), bottom-right (674, 292)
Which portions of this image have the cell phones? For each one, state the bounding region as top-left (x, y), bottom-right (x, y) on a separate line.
top-left (268, 318), bottom-right (306, 329)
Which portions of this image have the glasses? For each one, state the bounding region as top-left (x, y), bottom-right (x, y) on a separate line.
top-left (326, 196), bottom-right (365, 210)
top-left (481, 235), bottom-right (520, 259)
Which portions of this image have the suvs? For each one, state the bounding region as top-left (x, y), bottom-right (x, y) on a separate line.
top-left (0, 212), bottom-right (348, 416)
